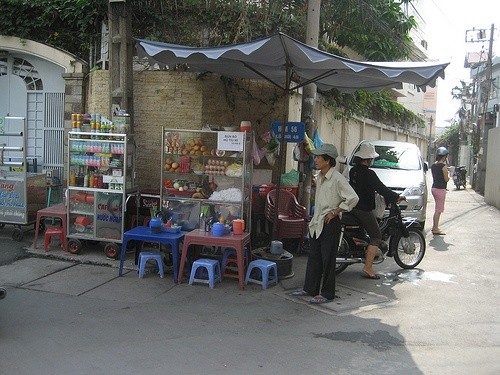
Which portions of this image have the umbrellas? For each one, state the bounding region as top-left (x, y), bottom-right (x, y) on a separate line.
top-left (131, 32), bottom-right (451, 246)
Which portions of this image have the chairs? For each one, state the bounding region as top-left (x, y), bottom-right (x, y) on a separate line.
top-left (263, 188), bottom-right (307, 254)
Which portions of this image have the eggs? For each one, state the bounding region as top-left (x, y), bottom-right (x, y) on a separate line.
top-left (204, 159), bottom-right (227, 176)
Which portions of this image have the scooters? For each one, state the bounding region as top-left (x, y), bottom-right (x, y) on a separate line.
top-left (452, 165), bottom-right (467, 190)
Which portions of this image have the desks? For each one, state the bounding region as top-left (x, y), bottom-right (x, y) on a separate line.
top-left (34, 203), bottom-right (68, 252)
top-left (178, 229), bottom-right (250, 292)
top-left (46, 184), bottom-right (62, 225)
top-left (119, 226), bottom-right (188, 284)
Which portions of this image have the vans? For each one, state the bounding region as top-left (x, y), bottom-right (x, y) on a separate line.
top-left (338, 139), bottom-right (429, 231)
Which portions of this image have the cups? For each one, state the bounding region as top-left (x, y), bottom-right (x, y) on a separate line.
top-left (75, 192), bottom-right (88, 203)
top-left (85, 194), bottom-right (94, 203)
top-left (233, 218), bottom-right (244, 234)
top-left (240, 121), bottom-right (251, 131)
top-left (76, 216), bottom-right (90, 226)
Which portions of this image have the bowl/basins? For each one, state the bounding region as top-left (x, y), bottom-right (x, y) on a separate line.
top-left (191, 167), bottom-right (206, 174)
top-left (182, 220), bottom-right (197, 230)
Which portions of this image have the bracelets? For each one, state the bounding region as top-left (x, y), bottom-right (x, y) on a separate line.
top-left (331, 210), bottom-right (336, 215)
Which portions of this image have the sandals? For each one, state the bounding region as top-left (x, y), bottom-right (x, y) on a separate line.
top-left (310, 295), bottom-right (333, 304)
top-left (292, 290), bottom-right (308, 296)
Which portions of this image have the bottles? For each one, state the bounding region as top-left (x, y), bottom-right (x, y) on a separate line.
top-left (150, 218), bottom-right (160, 233)
top-left (226, 210), bottom-right (232, 224)
top-left (174, 223), bottom-right (178, 228)
top-left (69, 166), bottom-right (108, 189)
top-left (270, 239), bottom-right (282, 255)
top-left (166, 217), bottom-right (173, 227)
top-left (212, 222), bottom-right (225, 236)
top-left (220, 216), bottom-right (225, 225)
top-left (70, 109), bottom-right (131, 169)
top-left (165, 177), bottom-right (203, 192)
top-left (450, 164), bottom-right (456, 177)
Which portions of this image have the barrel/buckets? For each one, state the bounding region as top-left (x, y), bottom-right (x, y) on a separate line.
top-left (180, 155), bottom-right (193, 173)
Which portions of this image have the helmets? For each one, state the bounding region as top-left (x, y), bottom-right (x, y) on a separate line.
top-left (436, 147), bottom-right (449, 157)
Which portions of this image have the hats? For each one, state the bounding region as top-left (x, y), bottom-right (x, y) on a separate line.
top-left (353, 143), bottom-right (380, 159)
top-left (311, 143), bottom-right (338, 159)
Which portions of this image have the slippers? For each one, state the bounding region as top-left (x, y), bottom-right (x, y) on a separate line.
top-left (361, 274), bottom-right (382, 280)
top-left (433, 230), bottom-right (446, 236)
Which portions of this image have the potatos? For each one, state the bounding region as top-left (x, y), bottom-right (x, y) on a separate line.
top-left (164, 157), bottom-right (180, 173)
top-left (182, 138), bottom-right (211, 155)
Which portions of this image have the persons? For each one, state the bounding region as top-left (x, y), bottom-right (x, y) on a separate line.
top-left (348, 143), bottom-right (407, 279)
top-left (431, 147), bottom-right (450, 235)
top-left (292, 144), bottom-right (359, 303)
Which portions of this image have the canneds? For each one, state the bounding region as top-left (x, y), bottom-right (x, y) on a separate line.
top-left (71, 113), bottom-right (101, 130)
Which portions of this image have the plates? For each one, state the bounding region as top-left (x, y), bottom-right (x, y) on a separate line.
top-left (224, 126), bottom-right (238, 131)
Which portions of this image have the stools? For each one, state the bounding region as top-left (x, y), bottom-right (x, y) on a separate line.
top-left (136, 251), bottom-right (165, 279)
top-left (44, 228), bottom-right (66, 252)
top-left (189, 247), bottom-right (278, 290)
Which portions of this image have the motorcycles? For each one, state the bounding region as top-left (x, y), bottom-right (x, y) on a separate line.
top-left (334, 198), bottom-right (426, 274)
